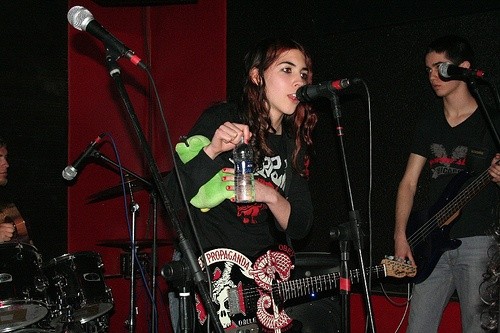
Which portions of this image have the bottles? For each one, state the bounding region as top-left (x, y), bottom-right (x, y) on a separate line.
top-left (233, 137), bottom-right (256, 204)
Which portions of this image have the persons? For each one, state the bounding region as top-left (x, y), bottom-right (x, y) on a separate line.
top-left (393, 37), bottom-right (500, 333)
top-left (0, 139), bottom-right (15, 243)
top-left (169, 38), bottom-right (318, 333)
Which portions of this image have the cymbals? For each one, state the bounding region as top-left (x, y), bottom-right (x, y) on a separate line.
top-left (96, 238), bottom-right (178, 252)
top-left (82, 170), bottom-right (172, 199)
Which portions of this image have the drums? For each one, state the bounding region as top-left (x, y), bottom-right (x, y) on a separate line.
top-left (46, 250), bottom-right (117, 325)
top-left (0, 240), bottom-right (50, 333)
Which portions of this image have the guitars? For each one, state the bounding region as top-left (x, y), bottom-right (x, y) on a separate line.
top-left (188, 246), bottom-right (418, 333)
top-left (405, 159), bottom-right (500, 284)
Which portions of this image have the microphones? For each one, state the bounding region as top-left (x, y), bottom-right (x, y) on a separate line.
top-left (439, 61), bottom-right (486, 78)
top-left (67, 6), bottom-right (149, 73)
top-left (296, 78), bottom-right (361, 103)
top-left (61, 134), bottom-right (105, 180)
